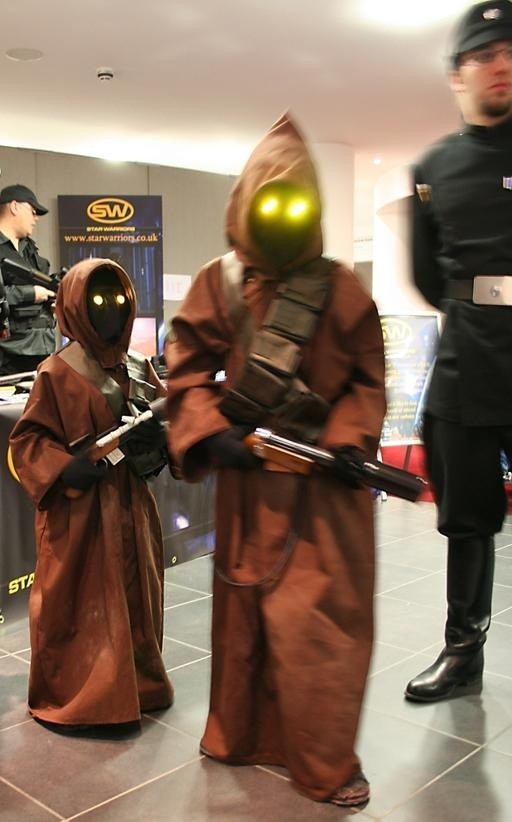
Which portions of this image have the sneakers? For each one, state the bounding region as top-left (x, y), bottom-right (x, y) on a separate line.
top-left (331, 773), bottom-right (369, 806)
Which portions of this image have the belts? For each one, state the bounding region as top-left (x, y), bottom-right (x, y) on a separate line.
top-left (441, 275), bottom-right (512, 308)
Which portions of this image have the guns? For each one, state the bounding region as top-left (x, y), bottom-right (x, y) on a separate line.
top-left (66, 396), bottom-right (168, 496)
top-left (241, 429), bottom-right (425, 503)
top-left (1, 257), bottom-right (70, 310)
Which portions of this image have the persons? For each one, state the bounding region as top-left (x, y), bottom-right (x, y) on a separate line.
top-left (159, 109), bottom-right (388, 809)
top-left (0, 184), bottom-right (58, 376)
top-left (405, 1), bottom-right (512, 703)
top-left (14, 257), bottom-right (175, 727)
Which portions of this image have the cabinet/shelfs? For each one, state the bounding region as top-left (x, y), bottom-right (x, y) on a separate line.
top-left (0, 343), bottom-right (213, 604)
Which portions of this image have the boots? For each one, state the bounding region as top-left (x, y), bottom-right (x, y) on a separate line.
top-left (406, 536), bottom-right (494, 701)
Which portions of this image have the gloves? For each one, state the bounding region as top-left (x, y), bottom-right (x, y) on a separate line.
top-left (203, 431), bottom-right (266, 468)
top-left (332, 447), bottom-right (360, 482)
top-left (60, 458), bottom-right (100, 491)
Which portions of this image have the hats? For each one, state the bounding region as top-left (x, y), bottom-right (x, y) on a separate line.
top-left (1, 185), bottom-right (48, 215)
top-left (456, 1), bottom-right (511, 54)
